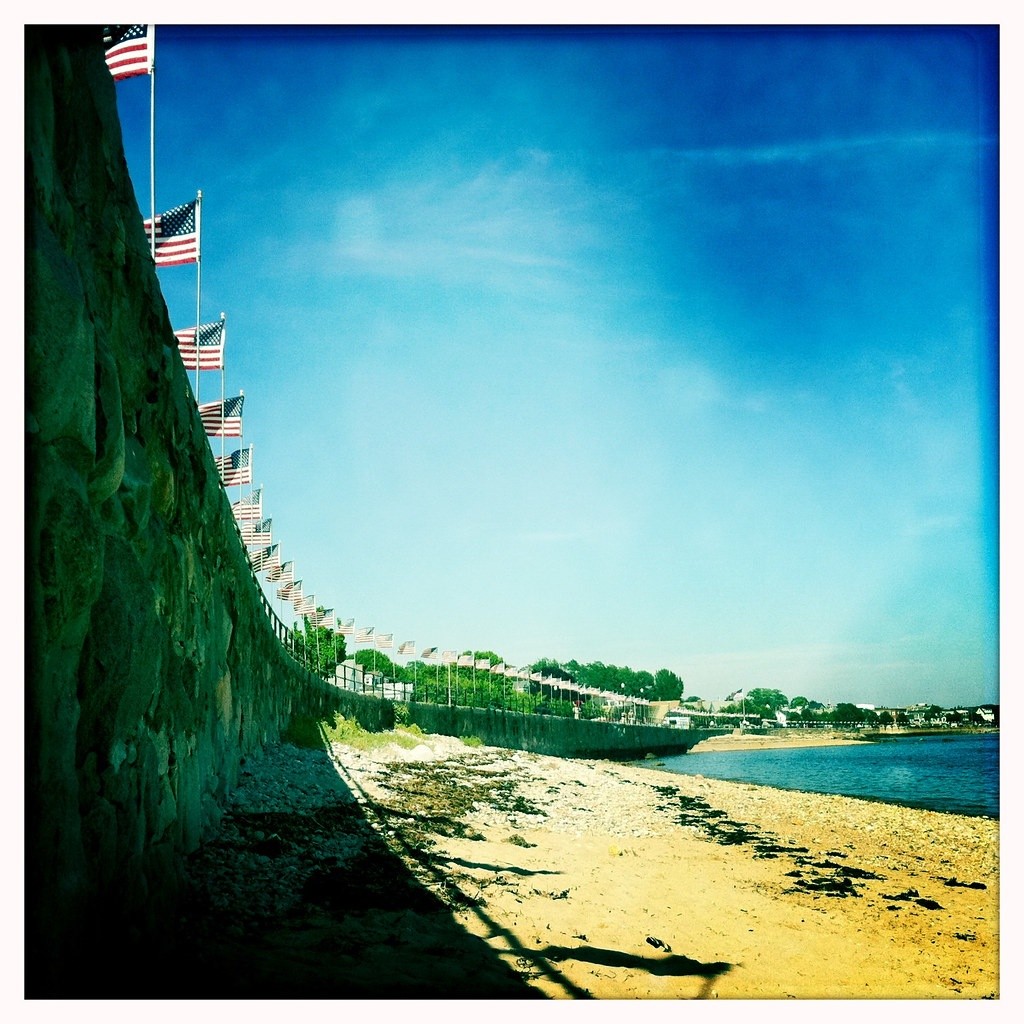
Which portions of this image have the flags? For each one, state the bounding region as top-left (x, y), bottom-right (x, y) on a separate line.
top-left (505, 667), bottom-right (649, 706)
top-left (198, 396), bottom-right (244, 437)
top-left (143, 199), bottom-right (201, 267)
top-left (277, 580), bottom-right (303, 601)
top-left (172, 320), bottom-right (224, 370)
top-left (240, 518), bottom-right (272, 545)
top-left (266, 561), bottom-right (293, 582)
top-left (294, 594), bottom-right (394, 649)
top-left (214, 448), bottom-right (249, 488)
top-left (668, 701), bottom-right (760, 718)
top-left (231, 489), bottom-right (263, 520)
top-left (248, 544), bottom-right (280, 573)
top-left (103, 25), bottom-right (155, 83)
top-left (397, 640), bottom-right (505, 674)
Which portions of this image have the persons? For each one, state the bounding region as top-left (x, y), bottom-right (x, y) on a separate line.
top-left (628, 709), bottom-right (633, 724)
top-left (621, 712), bottom-right (627, 724)
top-left (573, 700), bottom-right (585, 719)
